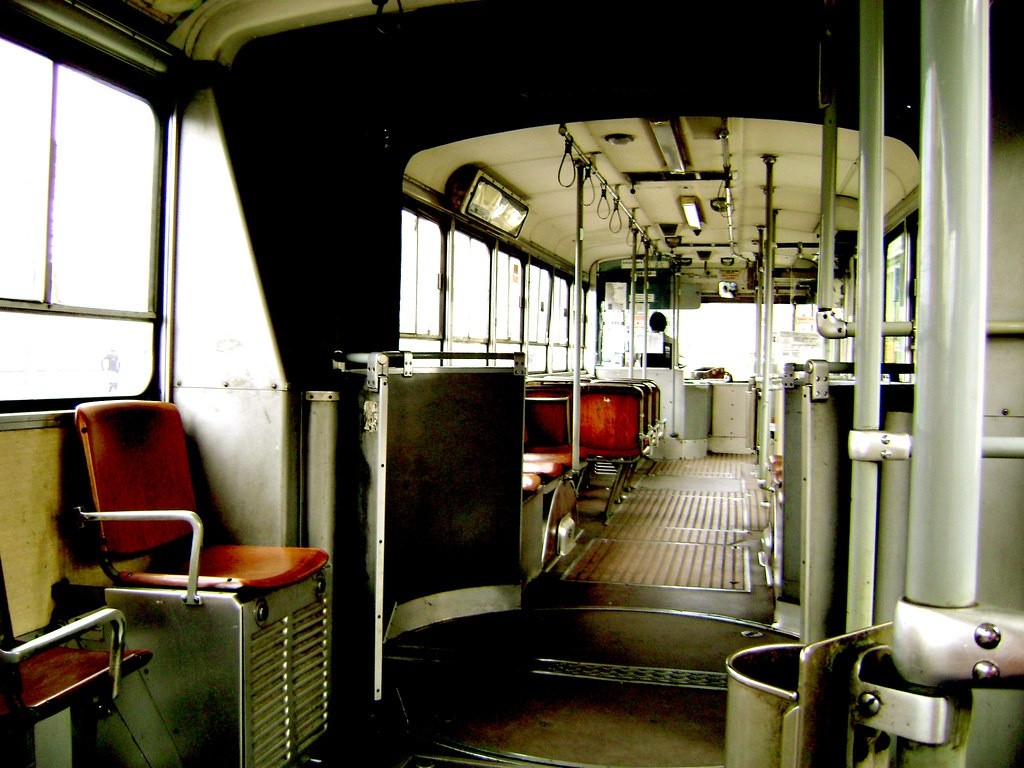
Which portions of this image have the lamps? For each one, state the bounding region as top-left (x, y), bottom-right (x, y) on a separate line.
top-left (681, 196), bottom-right (702, 230)
top-left (650, 116), bottom-right (688, 174)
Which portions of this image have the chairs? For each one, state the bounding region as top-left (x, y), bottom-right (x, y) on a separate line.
top-left (640, 342), bottom-right (672, 370)
top-left (522, 373), bottom-right (670, 573)
top-left (74, 400), bottom-right (330, 606)
top-left (0, 554), bottom-right (155, 768)
